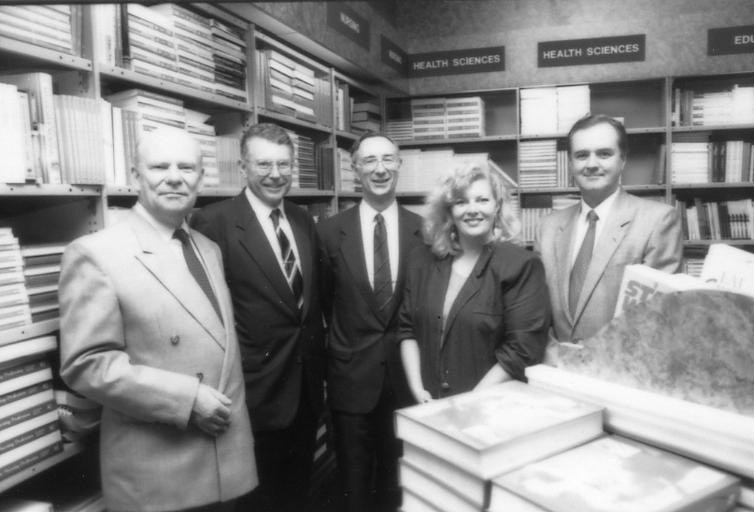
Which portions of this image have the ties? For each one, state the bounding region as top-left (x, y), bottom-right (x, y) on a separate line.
top-left (568, 212), bottom-right (608, 321)
top-left (172, 226), bottom-right (225, 326)
top-left (266, 209), bottom-right (304, 301)
top-left (373, 214), bottom-right (393, 317)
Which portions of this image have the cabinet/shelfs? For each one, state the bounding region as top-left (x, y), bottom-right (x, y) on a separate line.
top-left (10, 2), bottom-right (394, 512)
top-left (393, 70), bottom-right (751, 272)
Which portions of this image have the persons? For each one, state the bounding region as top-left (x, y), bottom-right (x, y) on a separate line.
top-left (58, 126), bottom-right (263, 512)
top-left (533, 114), bottom-right (685, 368)
top-left (397, 166), bottom-right (551, 408)
top-left (315, 132), bottom-right (430, 511)
top-left (190, 121), bottom-right (325, 511)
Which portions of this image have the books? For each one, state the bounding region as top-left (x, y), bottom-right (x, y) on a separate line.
top-left (122, 107), bottom-right (248, 194)
top-left (288, 137), bottom-right (384, 194)
top-left (671, 82), bottom-right (754, 278)
top-left (1, 1), bottom-right (385, 135)
top-left (0, 225), bottom-right (100, 511)
top-left (385, 95), bottom-right (516, 195)
top-left (393, 244), bottom-right (754, 511)
top-left (517, 87), bottom-right (592, 252)
top-left (0, 72), bottom-right (107, 194)
